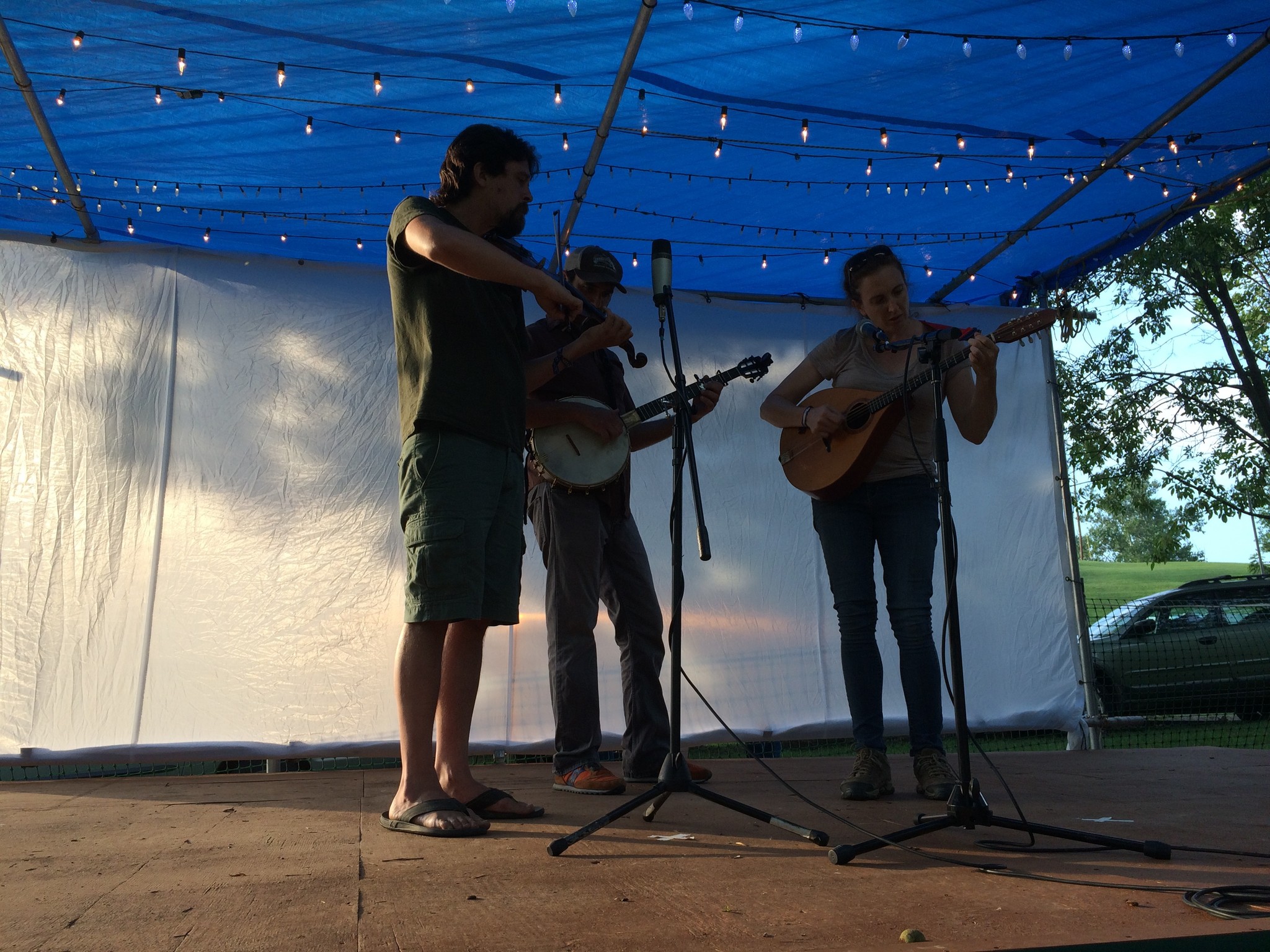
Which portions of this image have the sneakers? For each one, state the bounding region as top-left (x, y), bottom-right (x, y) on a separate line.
top-left (913, 747), bottom-right (960, 801)
top-left (552, 763), bottom-right (626, 795)
top-left (624, 761), bottom-right (713, 783)
top-left (839, 741), bottom-right (894, 799)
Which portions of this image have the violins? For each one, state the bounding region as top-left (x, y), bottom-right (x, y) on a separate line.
top-left (495, 231), bottom-right (649, 368)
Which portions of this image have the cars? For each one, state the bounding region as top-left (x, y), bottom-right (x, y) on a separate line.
top-left (1080, 574), bottom-right (1270, 727)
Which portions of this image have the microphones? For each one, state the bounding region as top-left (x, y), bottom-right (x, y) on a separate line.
top-left (855, 319), bottom-right (889, 342)
top-left (651, 239), bottom-right (672, 321)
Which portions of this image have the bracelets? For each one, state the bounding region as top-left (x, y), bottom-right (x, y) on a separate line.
top-left (552, 347), bottom-right (574, 374)
top-left (802, 406), bottom-right (813, 427)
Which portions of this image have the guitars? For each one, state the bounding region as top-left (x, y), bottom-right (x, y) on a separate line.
top-left (529, 353), bottom-right (774, 495)
top-left (779, 309), bottom-right (1061, 499)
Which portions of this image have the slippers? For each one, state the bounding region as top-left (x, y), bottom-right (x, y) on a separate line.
top-left (463, 787), bottom-right (544, 818)
top-left (379, 798), bottom-right (491, 837)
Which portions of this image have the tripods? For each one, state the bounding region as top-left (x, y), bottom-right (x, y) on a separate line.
top-left (827, 332), bottom-right (1172, 868)
top-left (546, 284), bottom-right (830, 856)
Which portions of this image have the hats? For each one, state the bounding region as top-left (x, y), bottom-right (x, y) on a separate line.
top-left (564, 245), bottom-right (623, 283)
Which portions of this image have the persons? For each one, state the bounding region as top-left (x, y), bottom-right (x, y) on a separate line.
top-left (759, 247), bottom-right (1003, 798)
top-left (524, 246), bottom-right (725, 795)
top-left (383, 123), bottom-right (636, 836)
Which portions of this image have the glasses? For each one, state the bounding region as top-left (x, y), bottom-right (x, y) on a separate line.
top-left (848, 245), bottom-right (897, 295)
